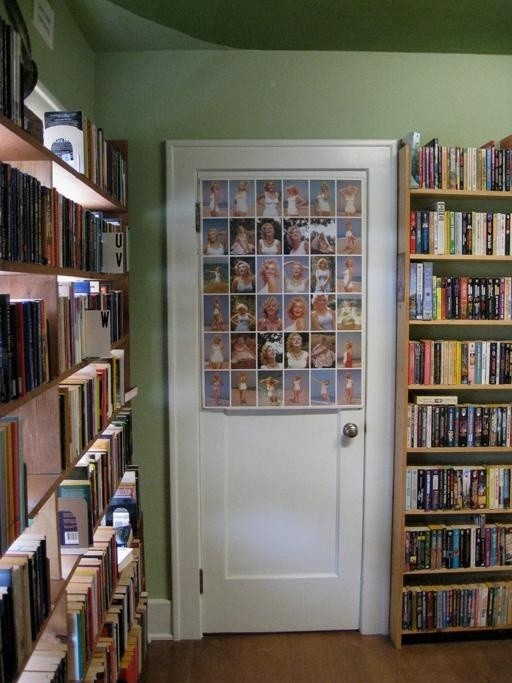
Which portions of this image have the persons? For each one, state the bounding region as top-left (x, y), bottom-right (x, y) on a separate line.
top-left (342, 258), bottom-right (353, 291)
top-left (258, 260), bottom-right (281, 292)
top-left (290, 375), bottom-right (302, 402)
top-left (283, 296), bottom-right (308, 332)
top-left (319, 232), bottom-right (334, 253)
top-left (344, 374), bottom-right (354, 403)
top-left (314, 182), bottom-right (331, 215)
top-left (237, 372), bottom-right (248, 404)
top-left (286, 333), bottom-right (308, 369)
top-left (257, 182), bottom-right (281, 216)
top-left (259, 296), bottom-right (282, 331)
top-left (342, 340), bottom-right (353, 368)
top-left (210, 373), bottom-right (224, 404)
top-left (312, 336), bottom-right (335, 368)
top-left (45, 110), bottom-right (129, 208)
top-left (341, 302), bottom-right (355, 326)
top-left (231, 304), bottom-right (256, 332)
top-left (0, 17), bottom-right (25, 131)
top-left (209, 182), bottom-right (222, 216)
top-left (232, 337), bottom-right (254, 365)
top-left (209, 336), bottom-right (225, 369)
top-left (259, 342), bottom-right (282, 369)
top-left (231, 225), bottom-right (254, 254)
top-left (283, 185), bottom-right (306, 216)
top-left (211, 297), bottom-right (225, 331)
top-left (208, 266), bottom-right (223, 284)
top-left (311, 294), bottom-right (335, 331)
top-left (315, 258), bottom-right (332, 292)
top-left (343, 222), bottom-right (358, 254)
top-left (339, 186), bottom-right (359, 216)
top-left (232, 182), bottom-right (250, 216)
top-left (232, 261), bottom-right (254, 292)
top-left (260, 377), bottom-right (280, 403)
top-left (312, 376), bottom-right (331, 403)
top-left (285, 226), bottom-right (309, 255)
top-left (203, 228), bottom-right (228, 255)
top-left (258, 222), bottom-right (280, 252)
top-left (283, 261), bottom-right (309, 292)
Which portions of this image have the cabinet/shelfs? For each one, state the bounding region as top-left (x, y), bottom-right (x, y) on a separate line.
top-left (2, 108), bottom-right (150, 683)
top-left (389, 131), bottom-right (512, 652)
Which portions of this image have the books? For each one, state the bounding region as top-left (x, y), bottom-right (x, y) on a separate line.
top-left (406, 404), bottom-right (511, 448)
top-left (402, 575), bottom-right (512, 629)
top-left (410, 201), bottom-right (512, 255)
top-left (0, 275), bottom-right (133, 474)
top-left (407, 339), bottom-right (512, 384)
top-left (1, 474), bottom-right (147, 683)
top-left (405, 514), bottom-right (512, 570)
top-left (414, 397), bottom-right (457, 406)
top-left (409, 261), bottom-right (512, 320)
top-left (406, 465), bottom-right (512, 510)
top-left (397, 131), bottom-right (512, 192)
top-left (0, 161), bottom-right (130, 273)
